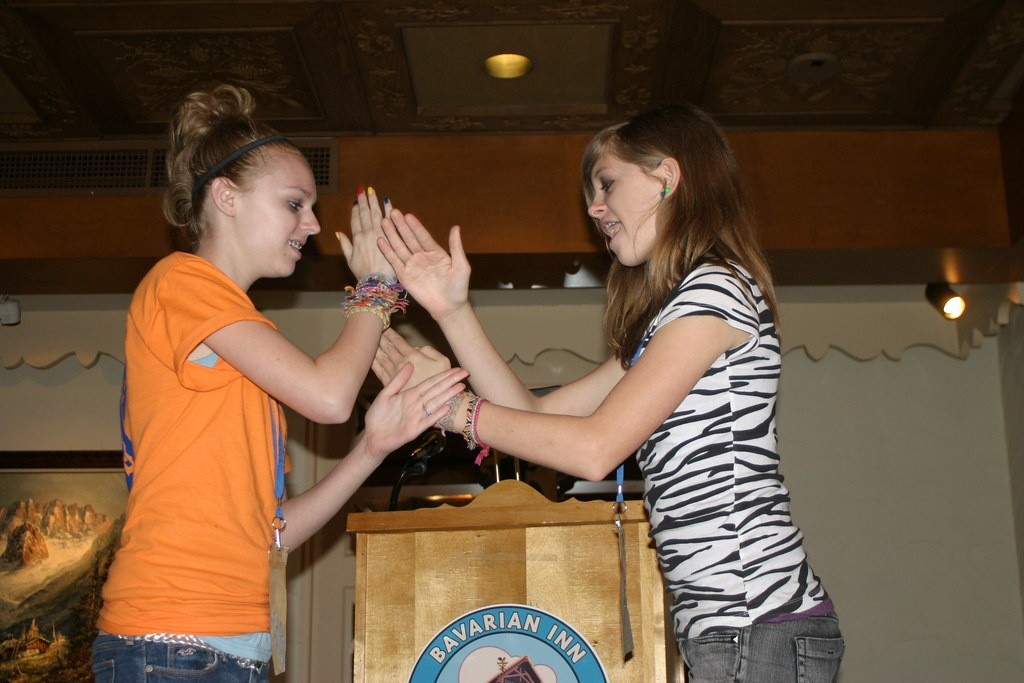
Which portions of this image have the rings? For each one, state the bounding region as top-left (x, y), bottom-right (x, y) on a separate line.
top-left (421, 402), bottom-right (432, 416)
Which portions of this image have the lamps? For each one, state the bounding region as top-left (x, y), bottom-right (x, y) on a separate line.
top-left (925, 278), bottom-right (967, 320)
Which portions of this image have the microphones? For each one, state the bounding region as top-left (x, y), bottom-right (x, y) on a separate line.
top-left (410, 430), bottom-right (447, 461)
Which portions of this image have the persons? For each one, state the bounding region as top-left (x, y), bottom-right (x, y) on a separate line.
top-left (369, 99), bottom-right (850, 683)
top-left (92, 85), bottom-right (476, 683)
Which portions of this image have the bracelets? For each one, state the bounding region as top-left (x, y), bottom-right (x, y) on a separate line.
top-left (437, 388), bottom-right (492, 466)
top-left (341, 270), bottom-right (410, 331)
